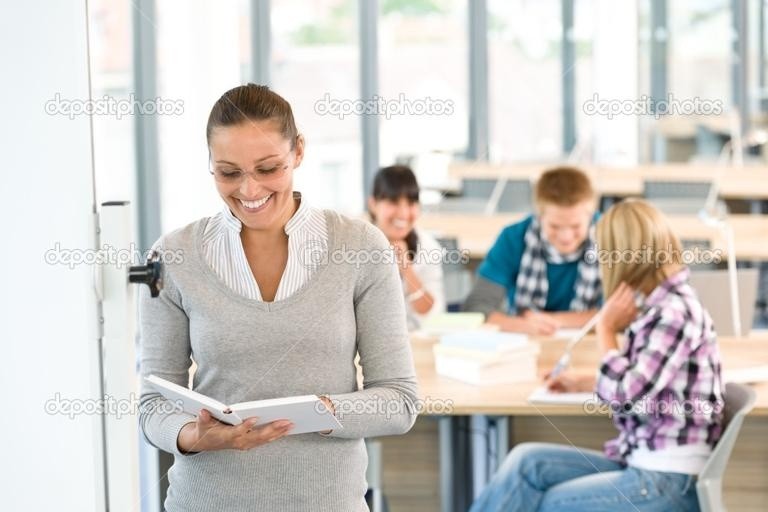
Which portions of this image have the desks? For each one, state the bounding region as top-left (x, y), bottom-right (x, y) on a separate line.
top-left (414, 108), bottom-right (767, 417)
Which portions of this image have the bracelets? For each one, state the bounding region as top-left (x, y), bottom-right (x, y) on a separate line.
top-left (405, 288), bottom-right (425, 300)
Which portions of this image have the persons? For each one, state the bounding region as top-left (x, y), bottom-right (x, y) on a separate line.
top-left (367, 166), bottom-right (445, 327)
top-left (468, 198), bottom-right (729, 512)
top-left (462, 167), bottom-right (604, 337)
top-left (136, 83), bottom-right (418, 511)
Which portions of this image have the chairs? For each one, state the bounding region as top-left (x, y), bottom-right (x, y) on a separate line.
top-left (695, 383), bottom-right (755, 512)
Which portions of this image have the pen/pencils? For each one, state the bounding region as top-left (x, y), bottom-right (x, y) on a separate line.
top-left (550, 353), bottom-right (570, 378)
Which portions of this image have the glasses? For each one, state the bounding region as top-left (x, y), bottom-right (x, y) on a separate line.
top-left (207, 144), bottom-right (297, 183)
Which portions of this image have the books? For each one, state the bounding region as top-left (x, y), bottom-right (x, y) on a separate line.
top-left (144, 374), bottom-right (342, 437)
